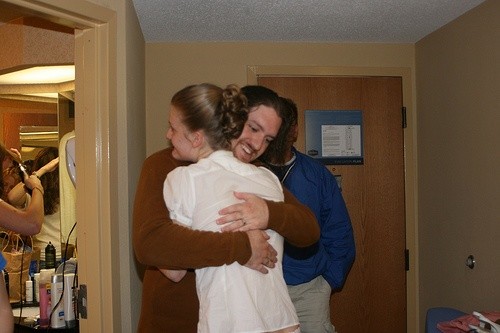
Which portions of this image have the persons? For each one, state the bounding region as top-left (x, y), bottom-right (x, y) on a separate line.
top-left (0, 143), bottom-right (60, 332)
top-left (131, 82), bottom-right (357, 333)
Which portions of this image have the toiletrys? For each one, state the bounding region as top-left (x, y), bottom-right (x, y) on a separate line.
top-left (23, 241), bottom-right (76, 329)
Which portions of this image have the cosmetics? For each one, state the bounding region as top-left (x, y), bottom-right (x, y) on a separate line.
top-left (25, 241), bottom-right (78, 330)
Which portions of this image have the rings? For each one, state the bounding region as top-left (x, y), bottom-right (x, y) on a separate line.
top-left (241, 218), bottom-right (247, 225)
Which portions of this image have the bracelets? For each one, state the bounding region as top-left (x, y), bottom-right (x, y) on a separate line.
top-left (33, 187), bottom-right (43, 194)
top-left (31, 171), bottom-right (40, 178)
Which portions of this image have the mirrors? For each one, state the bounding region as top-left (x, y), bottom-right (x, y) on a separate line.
top-left (0, 92), bottom-right (77, 269)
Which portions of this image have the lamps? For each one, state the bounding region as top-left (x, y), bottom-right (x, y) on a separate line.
top-left (0, 64), bottom-right (76, 84)
